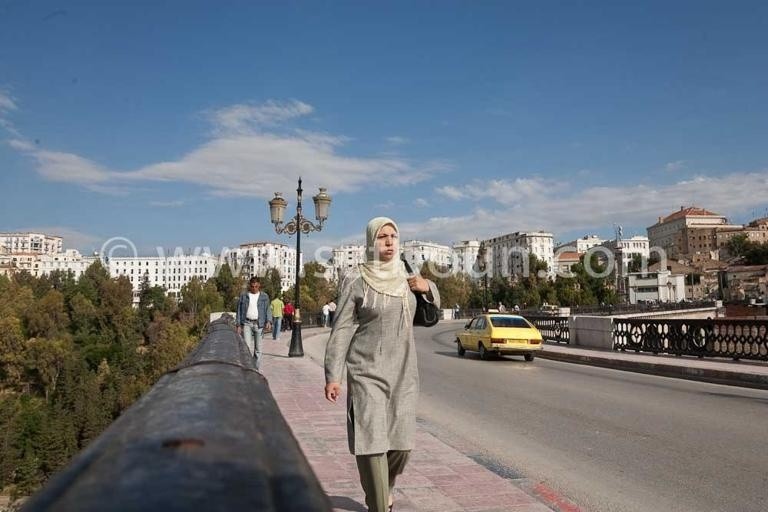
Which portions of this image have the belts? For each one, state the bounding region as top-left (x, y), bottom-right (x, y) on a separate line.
top-left (245, 318), bottom-right (258, 322)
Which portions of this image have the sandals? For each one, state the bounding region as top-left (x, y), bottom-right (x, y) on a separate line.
top-left (388, 502), bottom-right (393, 512)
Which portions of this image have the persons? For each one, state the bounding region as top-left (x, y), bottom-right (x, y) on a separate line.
top-left (454, 303), bottom-right (460, 320)
top-left (322, 300), bottom-right (337, 329)
top-left (270, 293), bottom-right (294, 340)
top-left (236, 276), bottom-right (273, 370)
top-left (497, 301), bottom-right (506, 312)
top-left (325, 216), bottom-right (441, 512)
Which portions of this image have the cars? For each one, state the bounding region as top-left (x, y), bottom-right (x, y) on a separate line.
top-left (454, 311), bottom-right (546, 363)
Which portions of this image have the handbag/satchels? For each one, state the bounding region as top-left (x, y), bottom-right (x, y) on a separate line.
top-left (402, 259), bottom-right (439, 327)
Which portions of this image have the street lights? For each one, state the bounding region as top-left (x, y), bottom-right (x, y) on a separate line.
top-left (269, 177), bottom-right (332, 356)
